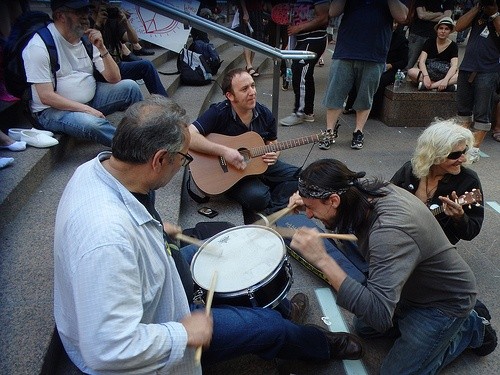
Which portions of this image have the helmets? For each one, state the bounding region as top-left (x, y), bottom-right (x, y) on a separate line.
top-left (200, 8), bottom-right (213, 19)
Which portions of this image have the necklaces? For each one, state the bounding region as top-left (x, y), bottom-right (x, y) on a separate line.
top-left (426, 175), bottom-right (437, 201)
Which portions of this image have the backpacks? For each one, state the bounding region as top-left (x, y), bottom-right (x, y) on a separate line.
top-left (2, 10), bottom-right (95, 99)
top-left (177, 44), bottom-right (212, 86)
top-left (189, 40), bottom-right (224, 76)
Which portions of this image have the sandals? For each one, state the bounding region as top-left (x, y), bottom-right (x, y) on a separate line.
top-left (246, 66), bottom-right (260, 77)
top-left (492, 128), bottom-right (500, 142)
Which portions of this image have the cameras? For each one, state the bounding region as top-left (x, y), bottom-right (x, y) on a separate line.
top-left (481, 0), bottom-right (491, 7)
top-left (104, 6), bottom-right (121, 19)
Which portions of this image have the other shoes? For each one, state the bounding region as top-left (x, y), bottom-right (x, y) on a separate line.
top-left (8, 128), bottom-right (53, 140)
top-left (343, 106), bottom-right (352, 114)
top-left (470, 147), bottom-right (480, 163)
top-left (121, 52), bottom-right (142, 61)
top-left (445, 83), bottom-right (457, 91)
top-left (473, 299), bottom-right (497, 356)
top-left (0, 89), bottom-right (21, 101)
top-left (318, 57), bottom-right (324, 66)
top-left (417, 81), bottom-right (431, 91)
top-left (20, 129), bottom-right (59, 148)
top-left (131, 47), bottom-right (155, 56)
top-left (282, 77), bottom-right (289, 91)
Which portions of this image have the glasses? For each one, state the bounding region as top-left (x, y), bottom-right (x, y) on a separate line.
top-left (446, 145), bottom-right (469, 160)
top-left (174, 150), bottom-right (194, 168)
top-left (61, 7), bottom-right (90, 15)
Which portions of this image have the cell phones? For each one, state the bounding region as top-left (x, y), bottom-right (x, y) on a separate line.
top-left (198, 207), bottom-right (219, 219)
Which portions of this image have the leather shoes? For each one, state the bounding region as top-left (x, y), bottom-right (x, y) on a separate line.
top-left (289, 292), bottom-right (309, 325)
top-left (304, 324), bottom-right (365, 361)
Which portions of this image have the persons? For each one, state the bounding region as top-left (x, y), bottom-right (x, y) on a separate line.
top-left (188, 68), bottom-right (304, 217)
top-left (389, 120), bottom-right (485, 245)
top-left (54, 95), bottom-right (366, 375)
top-left (0, 0), bottom-right (166, 168)
top-left (288, 159), bottom-right (496, 375)
top-left (192, 0), bottom-right (500, 149)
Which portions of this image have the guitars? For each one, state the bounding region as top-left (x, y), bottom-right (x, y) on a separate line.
top-left (427, 188), bottom-right (485, 218)
top-left (184, 127), bottom-right (336, 198)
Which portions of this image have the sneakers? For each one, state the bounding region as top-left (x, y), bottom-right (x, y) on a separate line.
top-left (297, 110), bottom-right (314, 122)
top-left (318, 129), bottom-right (335, 150)
top-left (280, 112), bottom-right (305, 125)
top-left (351, 130), bottom-right (365, 149)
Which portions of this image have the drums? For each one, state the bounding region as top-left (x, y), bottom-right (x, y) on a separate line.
top-left (187, 225), bottom-right (296, 312)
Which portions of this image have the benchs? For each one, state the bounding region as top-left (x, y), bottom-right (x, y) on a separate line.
top-left (378, 83), bottom-right (458, 127)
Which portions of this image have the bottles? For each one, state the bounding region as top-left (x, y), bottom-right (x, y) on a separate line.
top-left (394, 69), bottom-right (401, 87)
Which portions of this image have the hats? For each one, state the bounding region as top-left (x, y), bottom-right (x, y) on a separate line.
top-left (434, 17), bottom-right (456, 35)
top-left (50, 0), bottom-right (96, 11)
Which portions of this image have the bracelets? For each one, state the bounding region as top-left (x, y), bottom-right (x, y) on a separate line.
top-left (99, 51), bottom-right (109, 58)
